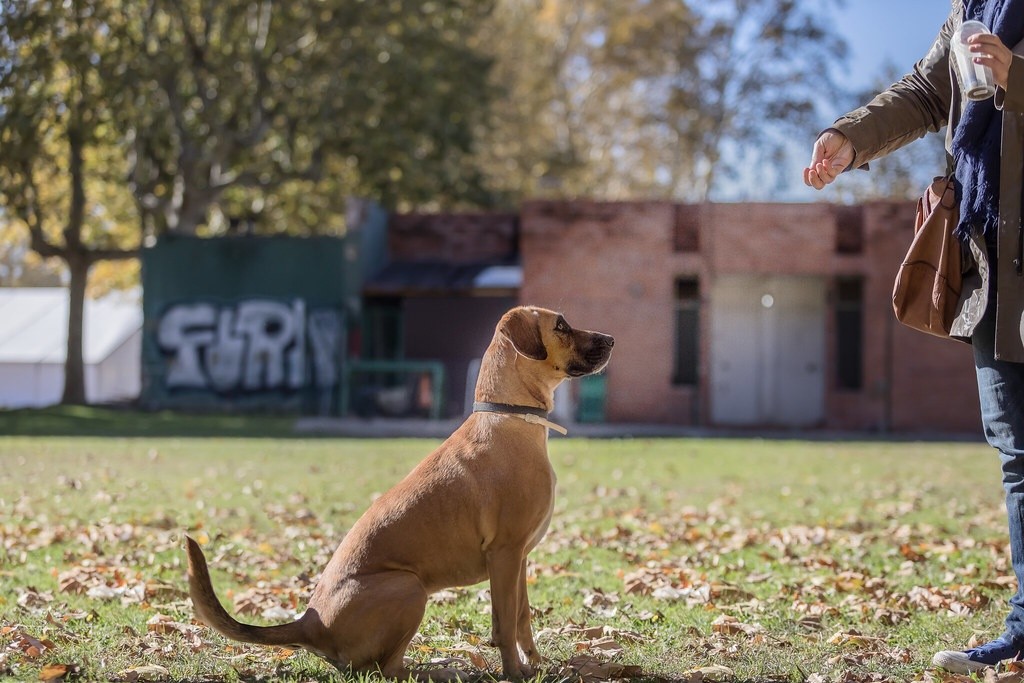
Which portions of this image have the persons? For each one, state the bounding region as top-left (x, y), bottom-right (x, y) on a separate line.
top-left (800, 1), bottom-right (1024, 677)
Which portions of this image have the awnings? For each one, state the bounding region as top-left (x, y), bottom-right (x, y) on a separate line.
top-left (364, 259), bottom-right (524, 303)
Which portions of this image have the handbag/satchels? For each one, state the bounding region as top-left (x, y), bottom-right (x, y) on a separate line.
top-left (892, 174), bottom-right (972, 345)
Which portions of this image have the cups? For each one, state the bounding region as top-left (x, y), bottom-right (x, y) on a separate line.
top-left (951, 21), bottom-right (995, 99)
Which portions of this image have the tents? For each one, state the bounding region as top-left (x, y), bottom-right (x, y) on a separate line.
top-left (0, 287), bottom-right (143, 410)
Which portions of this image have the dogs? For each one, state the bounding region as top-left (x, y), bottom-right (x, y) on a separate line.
top-left (183, 306), bottom-right (615, 683)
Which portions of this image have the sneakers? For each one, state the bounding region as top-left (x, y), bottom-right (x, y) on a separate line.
top-left (933, 638), bottom-right (1024, 674)
